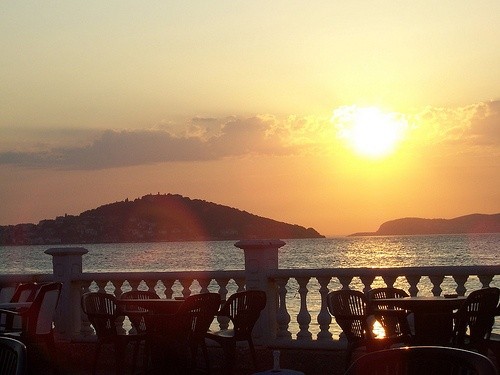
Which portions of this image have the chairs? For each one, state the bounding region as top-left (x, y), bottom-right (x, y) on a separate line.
top-left (0, 282), bottom-right (63, 375)
top-left (326, 287), bottom-right (500, 375)
top-left (81, 291), bottom-right (267, 375)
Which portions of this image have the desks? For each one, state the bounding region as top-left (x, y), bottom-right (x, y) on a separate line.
top-left (119, 298), bottom-right (186, 313)
top-left (370, 296), bottom-right (468, 346)
top-left (0, 302), bottom-right (33, 308)
